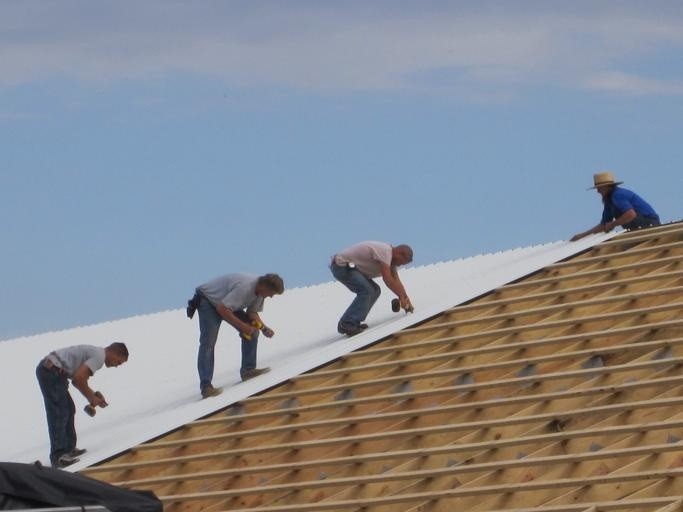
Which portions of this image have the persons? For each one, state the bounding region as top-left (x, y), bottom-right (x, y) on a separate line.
top-left (191, 272), bottom-right (284, 403)
top-left (566, 171), bottom-right (661, 243)
top-left (34, 341), bottom-right (129, 469)
top-left (329, 240), bottom-right (416, 336)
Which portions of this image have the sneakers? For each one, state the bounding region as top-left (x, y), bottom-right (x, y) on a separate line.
top-left (242, 367), bottom-right (270, 382)
top-left (52, 447), bottom-right (86, 469)
top-left (338, 320), bottom-right (368, 336)
top-left (202, 384), bottom-right (224, 398)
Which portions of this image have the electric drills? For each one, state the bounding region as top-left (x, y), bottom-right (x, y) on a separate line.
top-left (392, 299), bottom-right (415, 314)
top-left (83, 391), bottom-right (109, 417)
top-left (239, 319), bottom-right (267, 341)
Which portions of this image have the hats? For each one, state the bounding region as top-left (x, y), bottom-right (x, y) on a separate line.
top-left (585, 172), bottom-right (623, 191)
